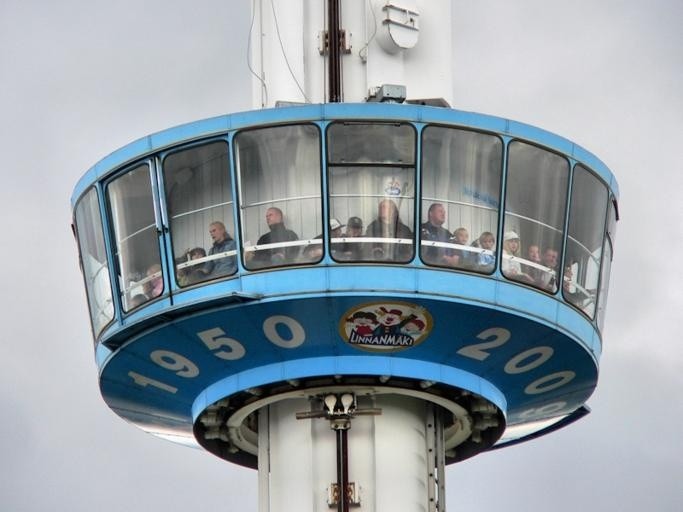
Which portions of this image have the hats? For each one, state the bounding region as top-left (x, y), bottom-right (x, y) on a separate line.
top-left (329, 218), bottom-right (345, 232)
top-left (347, 217), bottom-right (363, 229)
top-left (503, 230), bottom-right (520, 241)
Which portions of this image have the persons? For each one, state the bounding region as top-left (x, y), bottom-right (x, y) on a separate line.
top-left (175, 220), bottom-right (238, 286)
top-left (303, 199), bottom-right (414, 261)
top-left (422, 203), bottom-right (496, 271)
top-left (501, 231), bottom-right (599, 301)
top-left (130, 264), bottom-right (164, 309)
top-left (246, 207), bottom-right (300, 270)
